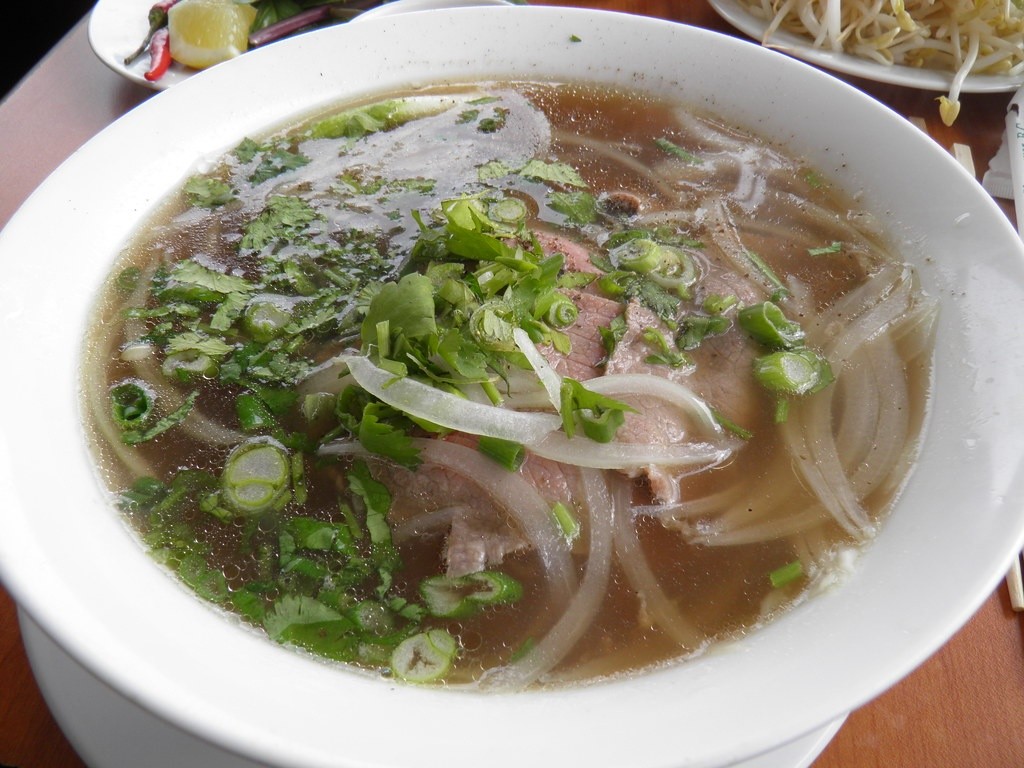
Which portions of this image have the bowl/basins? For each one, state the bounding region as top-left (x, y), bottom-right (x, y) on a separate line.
top-left (0, 4), bottom-right (1024, 767)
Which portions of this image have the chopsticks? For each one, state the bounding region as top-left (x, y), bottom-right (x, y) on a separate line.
top-left (908, 115), bottom-right (1024, 613)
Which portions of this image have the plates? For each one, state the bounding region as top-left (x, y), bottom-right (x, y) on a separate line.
top-left (708, 0), bottom-right (1024, 95)
top-left (14, 602), bottom-right (851, 767)
top-left (87, 1), bottom-right (203, 91)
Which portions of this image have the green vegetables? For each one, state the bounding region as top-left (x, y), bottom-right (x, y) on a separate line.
top-left (98, 89), bottom-right (853, 683)
